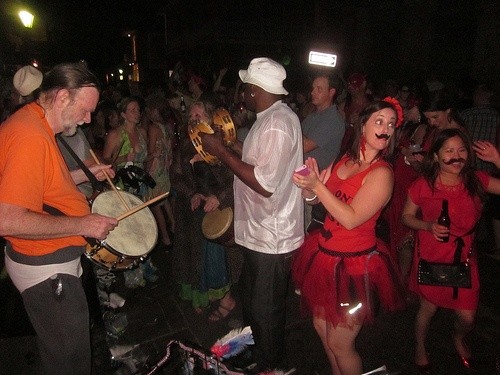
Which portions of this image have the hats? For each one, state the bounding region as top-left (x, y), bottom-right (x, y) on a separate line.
top-left (12, 64), bottom-right (50, 104)
top-left (239, 57), bottom-right (290, 95)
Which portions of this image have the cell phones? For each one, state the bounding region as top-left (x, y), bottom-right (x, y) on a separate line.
top-left (294, 165), bottom-right (310, 176)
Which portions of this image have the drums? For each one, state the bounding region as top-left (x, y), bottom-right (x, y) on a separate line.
top-left (203, 203), bottom-right (243, 251)
top-left (83, 188), bottom-right (159, 272)
top-left (186, 102), bottom-right (237, 167)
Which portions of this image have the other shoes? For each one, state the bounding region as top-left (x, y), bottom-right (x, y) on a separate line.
top-left (454, 341), bottom-right (480, 373)
top-left (414, 351), bottom-right (433, 375)
top-left (230, 349), bottom-right (258, 372)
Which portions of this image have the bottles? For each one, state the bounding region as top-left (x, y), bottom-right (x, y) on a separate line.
top-left (180, 95), bottom-right (186, 113)
top-left (438, 200), bottom-right (450, 242)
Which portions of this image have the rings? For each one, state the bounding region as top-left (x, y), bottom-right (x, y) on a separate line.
top-left (481, 146), bottom-right (486, 150)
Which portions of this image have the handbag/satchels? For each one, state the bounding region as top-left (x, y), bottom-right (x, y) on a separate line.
top-left (418, 259), bottom-right (472, 289)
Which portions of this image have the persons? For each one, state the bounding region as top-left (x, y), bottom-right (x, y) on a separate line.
top-left (292, 101), bottom-right (408, 374)
top-left (36, 55), bottom-right (150, 366)
top-left (401, 128), bottom-right (500, 375)
top-left (0, 62), bottom-right (120, 375)
top-left (196, 54), bottom-right (307, 375)
top-left (174, 99), bottom-right (239, 322)
top-left (0, 48), bottom-right (500, 306)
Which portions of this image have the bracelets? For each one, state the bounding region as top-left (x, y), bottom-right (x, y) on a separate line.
top-left (300, 192), bottom-right (319, 203)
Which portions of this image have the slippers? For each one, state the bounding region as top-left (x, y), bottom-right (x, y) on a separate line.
top-left (206, 300), bottom-right (239, 324)
top-left (192, 303), bottom-right (212, 315)
